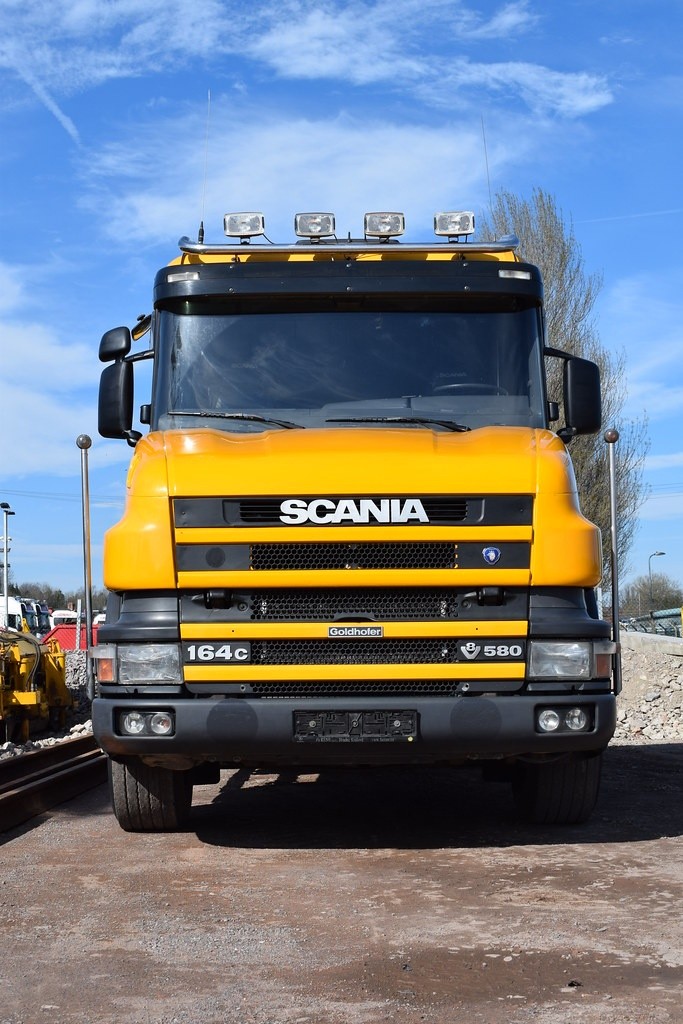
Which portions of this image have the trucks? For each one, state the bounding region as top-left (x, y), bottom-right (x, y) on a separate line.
top-left (85, 209), bottom-right (625, 839)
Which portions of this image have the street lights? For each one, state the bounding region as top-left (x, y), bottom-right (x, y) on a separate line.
top-left (648, 551), bottom-right (665, 621)
top-left (0, 502), bottom-right (16, 626)
